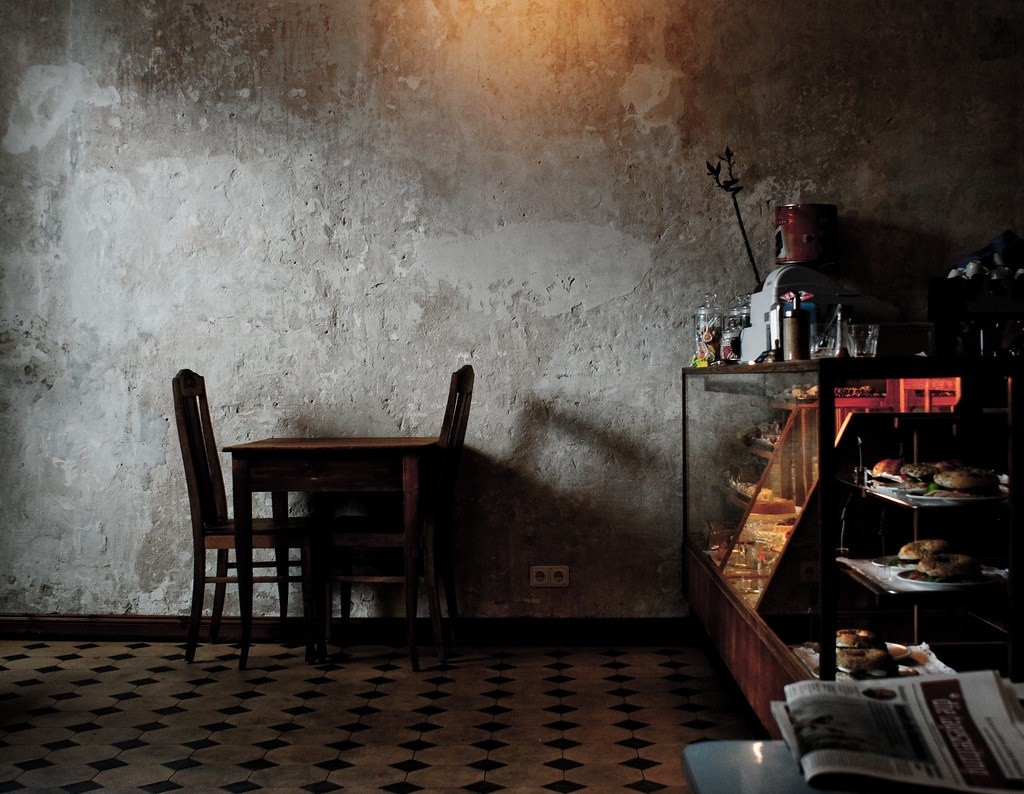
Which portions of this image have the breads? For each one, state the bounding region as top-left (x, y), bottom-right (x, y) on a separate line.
top-left (897, 538), bottom-right (950, 560)
top-left (917, 553), bottom-right (982, 577)
top-left (836, 628), bottom-right (900, 678)
top-left (871, 457), bottom-right (1002, 498)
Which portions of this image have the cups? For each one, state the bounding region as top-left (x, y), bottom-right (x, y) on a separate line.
top-left (810, 324), bottom-right (842, 360)
top-left (845, 324), bottom-right (880, 357)
top-left (947, 260), bottom-right (998, 279)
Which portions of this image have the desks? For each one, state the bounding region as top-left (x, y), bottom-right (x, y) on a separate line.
top-left (223, 437), bottom-right (439, 671)
top-left (681, 740), bottom-right (824, 794)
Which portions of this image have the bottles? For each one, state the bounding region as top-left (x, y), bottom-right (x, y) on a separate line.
top-left (691, 292), bottom-right (751, 361)
top-left (783, 293), bottom-right (809, 361)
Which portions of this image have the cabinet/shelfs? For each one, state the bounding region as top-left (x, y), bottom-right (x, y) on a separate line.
top-left (681, 359), bottom-right (1024, 740)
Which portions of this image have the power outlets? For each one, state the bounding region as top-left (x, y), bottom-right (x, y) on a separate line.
top-left (530, 566), bottom-right (570, 587)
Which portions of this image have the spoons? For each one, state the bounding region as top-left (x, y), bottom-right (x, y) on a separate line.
top-left (810, 303), bottom-right (842, 354)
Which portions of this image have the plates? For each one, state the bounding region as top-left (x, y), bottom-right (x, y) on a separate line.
top-left (870, 557), bottom-right (917, 572)
top-left (895, 569), bottom-right (991, 588)
top-left (904, 489), bottom-right (1008, 507)
top-left (811, 665), bottom-right (920, 679)
top-left (885, 642), bottom-right (911, 659)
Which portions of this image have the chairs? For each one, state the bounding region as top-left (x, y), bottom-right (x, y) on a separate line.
top-left (317, 364), bottom-right (475, 671)
top-left (173, 368), bottom-right (317, 665)
